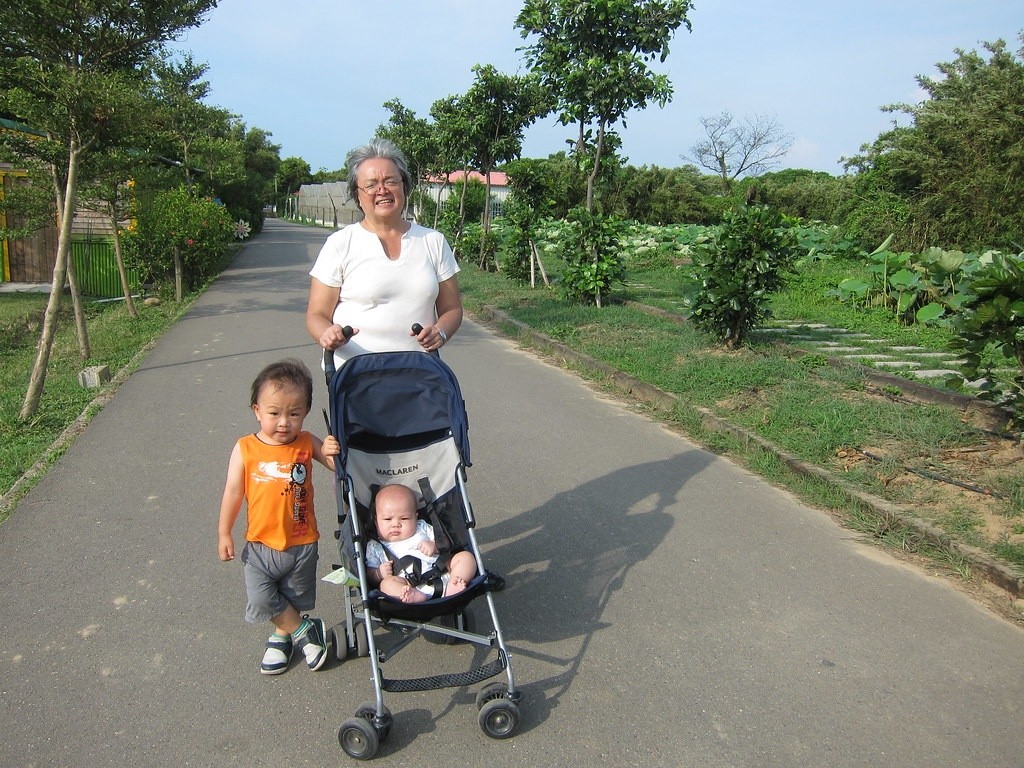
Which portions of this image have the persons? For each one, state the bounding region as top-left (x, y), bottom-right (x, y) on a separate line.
top-left (218, 362), bottom-right (340, 675)
top-left (306, 139), bottom-right (507, 593)
top-left (365, 484), bottom-right (476, 603)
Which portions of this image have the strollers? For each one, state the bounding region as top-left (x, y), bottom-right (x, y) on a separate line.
top-left (322, 323), bottom-right (519, 760)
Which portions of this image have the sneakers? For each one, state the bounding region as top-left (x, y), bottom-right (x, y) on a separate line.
top-left (294, 614), bottom-right (327, 672)
top-left (261, 637), bottom-right (294, 674)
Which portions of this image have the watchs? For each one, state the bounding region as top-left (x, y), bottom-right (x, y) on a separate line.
top-left (434, 326), bottom-right (447, 344)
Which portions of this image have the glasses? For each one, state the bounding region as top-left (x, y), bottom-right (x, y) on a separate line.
top-left (357, 177), bottom-right (402, 195)
